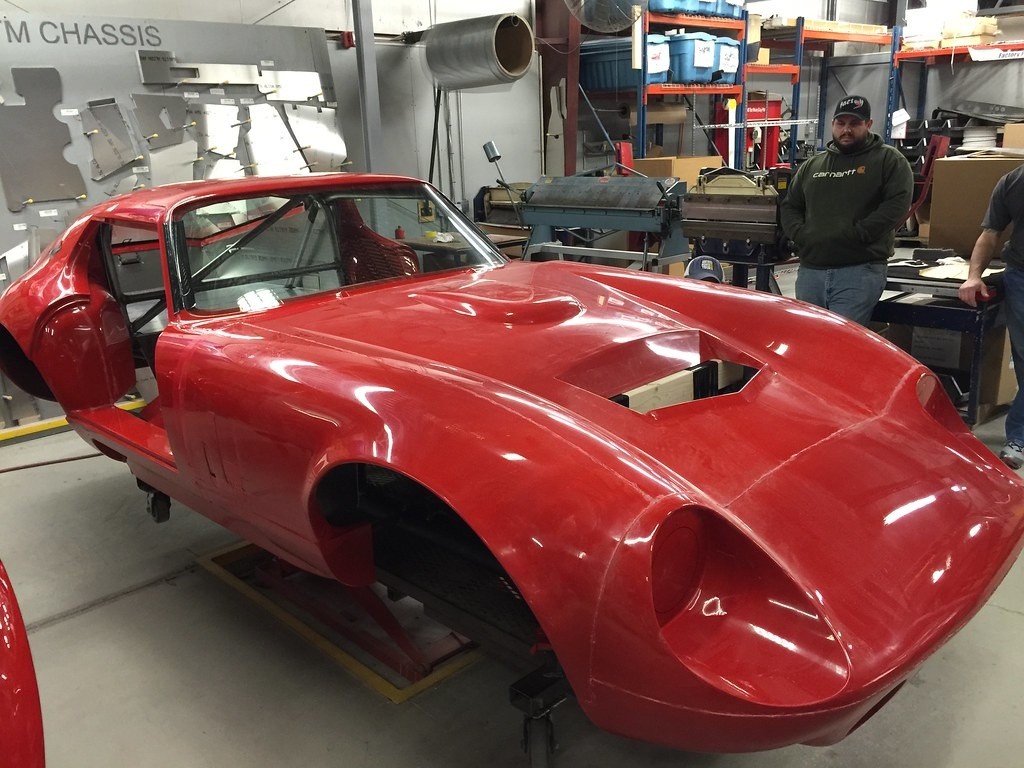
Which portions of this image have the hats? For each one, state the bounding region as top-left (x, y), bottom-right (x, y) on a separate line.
top-left (833, 95), bottom-right (871, 121)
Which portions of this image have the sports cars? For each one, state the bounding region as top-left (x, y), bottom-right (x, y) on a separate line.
top-left (1, 175), bottom-right (1024, 757)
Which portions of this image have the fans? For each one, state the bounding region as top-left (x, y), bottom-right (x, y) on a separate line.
top-left (561, 0), bottom-right (649, 34)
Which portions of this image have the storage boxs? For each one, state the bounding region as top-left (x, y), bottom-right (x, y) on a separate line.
top-left (709, 36), bottom-right (739, 85)
top-left (579, 33), bottom-right (670, 92)
top-left (907, 150), bottom-right (1023, 406)
top-left (630, 151), bottom-right (724, 191)
top-left (664, 32), bottom-right (716, 85)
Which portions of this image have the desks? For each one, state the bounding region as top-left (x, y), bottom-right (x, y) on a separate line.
top-left (390, 226), bottom-right (535, 271)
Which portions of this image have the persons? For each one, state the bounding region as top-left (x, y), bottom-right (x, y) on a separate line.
top-left (779, 95), bottom-right (913, 326)
top-left (958, 164), bottom-right (1024, 469)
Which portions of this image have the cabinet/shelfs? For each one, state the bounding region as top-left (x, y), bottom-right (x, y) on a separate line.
top-left (539, 0), bottom-right (1023, 198)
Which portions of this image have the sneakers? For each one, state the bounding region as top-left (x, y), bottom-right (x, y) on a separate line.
top-left (1000, 441), bottom-right (1024, 469)
